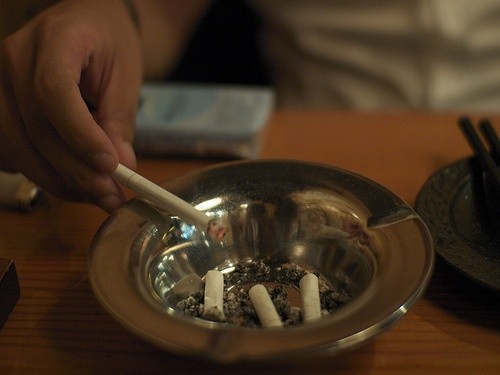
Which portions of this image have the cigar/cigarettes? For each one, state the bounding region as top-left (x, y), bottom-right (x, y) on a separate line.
top-left (112, 164), bottom-right (229, 240)
top-left (201, 269), bottom-right (320, 330)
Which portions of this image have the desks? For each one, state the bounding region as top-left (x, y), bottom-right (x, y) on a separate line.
top-left (1, 110), bottom-right (500, 375)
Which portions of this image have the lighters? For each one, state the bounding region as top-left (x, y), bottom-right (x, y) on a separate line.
top-left (0, 171), bottom-right (44, 213)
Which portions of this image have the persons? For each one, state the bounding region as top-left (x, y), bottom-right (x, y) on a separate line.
top-left (0, 0), bottom-right (500, 217)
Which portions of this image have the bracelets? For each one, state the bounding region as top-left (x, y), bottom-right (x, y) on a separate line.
top-left (125, 0), bottom-right (141, 32)
